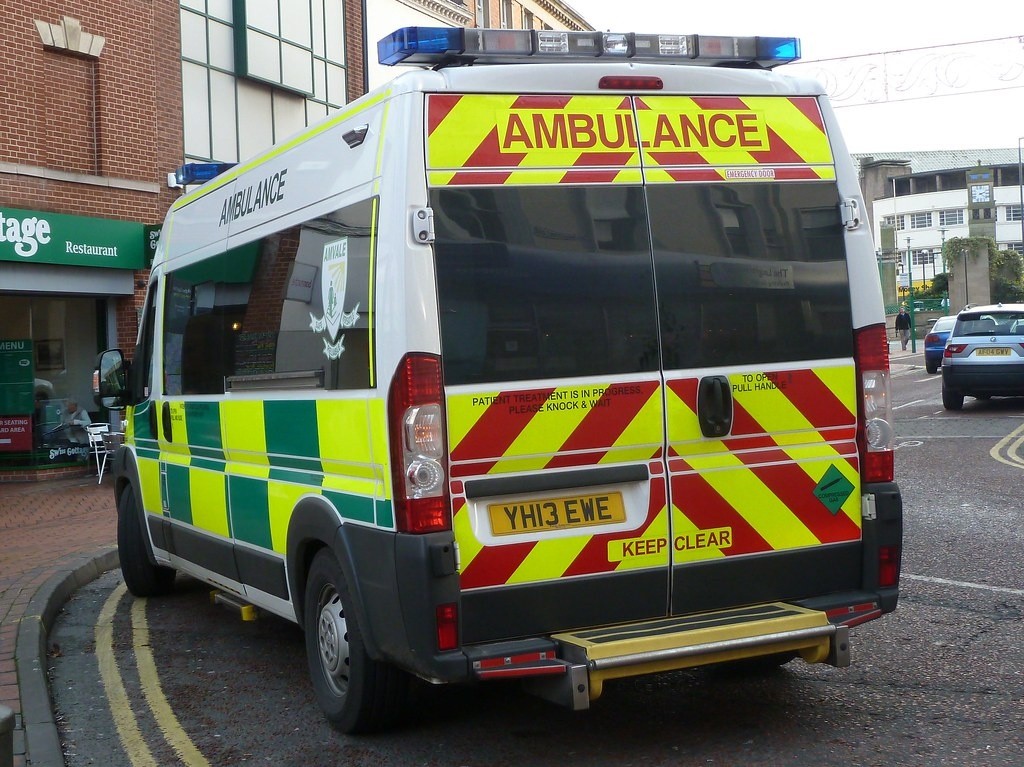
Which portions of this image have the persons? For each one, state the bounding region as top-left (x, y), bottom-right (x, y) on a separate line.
top-left (895, 307), bottom-right (911, 351)
top-left (33, 391), bottom-right (51, 447)
top-left (63, 398), bottom-right (91, 444)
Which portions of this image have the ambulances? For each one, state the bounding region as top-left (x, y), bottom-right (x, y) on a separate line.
top-left (92, 25), bottom-right (908, 737)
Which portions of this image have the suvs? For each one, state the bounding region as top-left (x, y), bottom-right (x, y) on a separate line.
top-left (937, 300), bottom-right (1024, 412)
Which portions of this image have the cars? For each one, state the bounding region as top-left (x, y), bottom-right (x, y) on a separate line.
top-left (924, 314), bottom-right (999, 374)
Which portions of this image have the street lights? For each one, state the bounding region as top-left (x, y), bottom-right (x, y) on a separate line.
top-left (937, 227), bottom-right (950, 315)
top-left (902, 236), bottom-right (917, 353)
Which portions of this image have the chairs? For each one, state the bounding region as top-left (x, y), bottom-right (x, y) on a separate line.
top-left (86, 422), bottom-right (125, 485)
